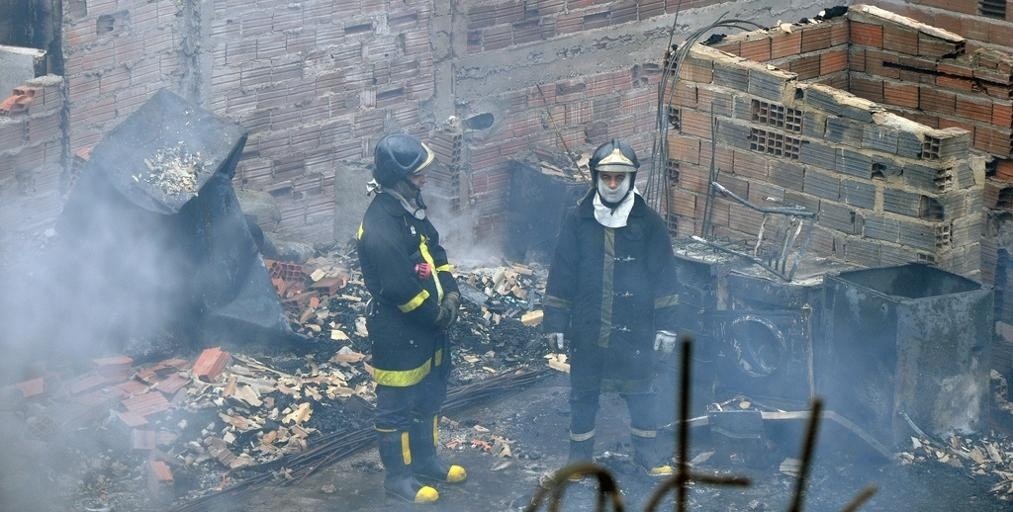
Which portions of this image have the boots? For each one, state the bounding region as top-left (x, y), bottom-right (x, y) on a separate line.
top-left (564, 429), bottom-right (595, 481)
top-left (412, 413), bottom-right (467, 483)
top-left (630, 428), bottom-right (673, 477)
top-left (377, 427), bottom-right (439, 503)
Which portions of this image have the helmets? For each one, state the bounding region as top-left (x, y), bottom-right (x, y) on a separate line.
top-left (372, 133), bottom-right (434, 184)
top-left (589, 138), bottom-right (640, 191)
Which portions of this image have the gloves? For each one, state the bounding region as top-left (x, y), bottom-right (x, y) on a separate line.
top-left (653, 333), bottom-right (677, 363)
top-left (434, 291), bottom-right (460, 330)
top-left (548, 332), bottom-right (564, 352)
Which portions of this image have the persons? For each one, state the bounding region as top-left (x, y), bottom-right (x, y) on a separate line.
top-left (541, 139), bottom-right (679, 486)
top-left (356, 133), bottom-right (467, 504)
